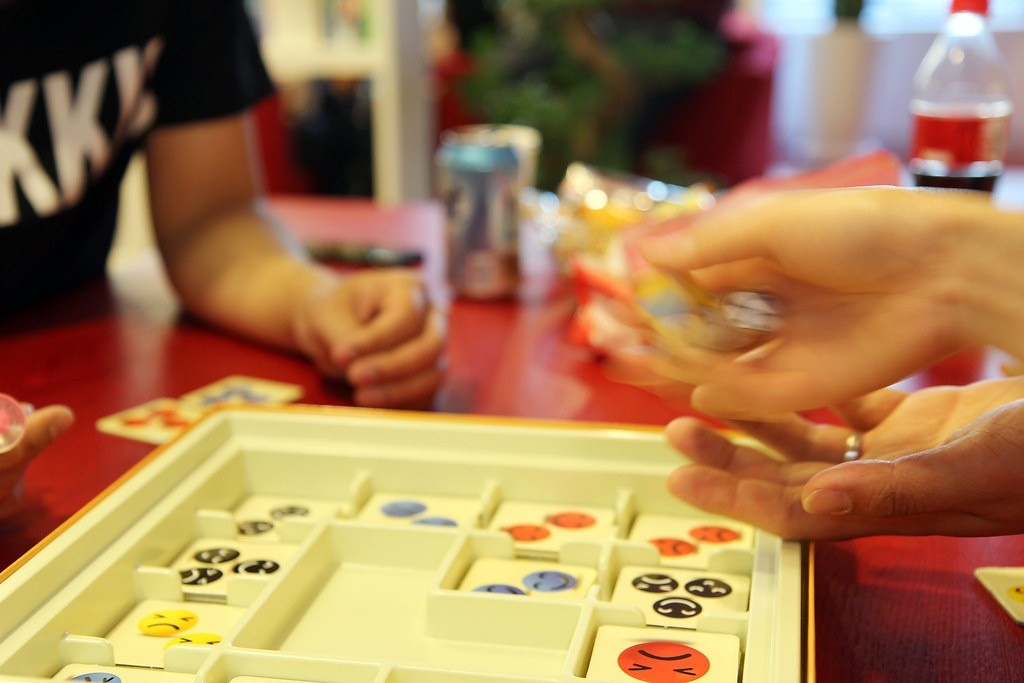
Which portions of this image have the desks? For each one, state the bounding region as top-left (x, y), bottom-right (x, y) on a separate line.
top-left (0, 195), bottom-right (1024, 683)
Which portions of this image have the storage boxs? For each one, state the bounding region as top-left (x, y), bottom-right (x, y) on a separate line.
top-left (29, 404), bottom-right (814, 682)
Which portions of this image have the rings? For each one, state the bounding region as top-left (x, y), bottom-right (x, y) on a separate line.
top-left (844, 431), bottom-right (862, 460)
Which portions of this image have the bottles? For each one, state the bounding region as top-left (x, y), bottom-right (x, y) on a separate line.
top-left (909, 0), bottom-right (1013, 200)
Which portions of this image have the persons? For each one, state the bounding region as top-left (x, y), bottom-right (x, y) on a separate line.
top-left (0, 0), bottom-right (445, 487)
top-left (580, 189), bottom-right (1024, 543)
